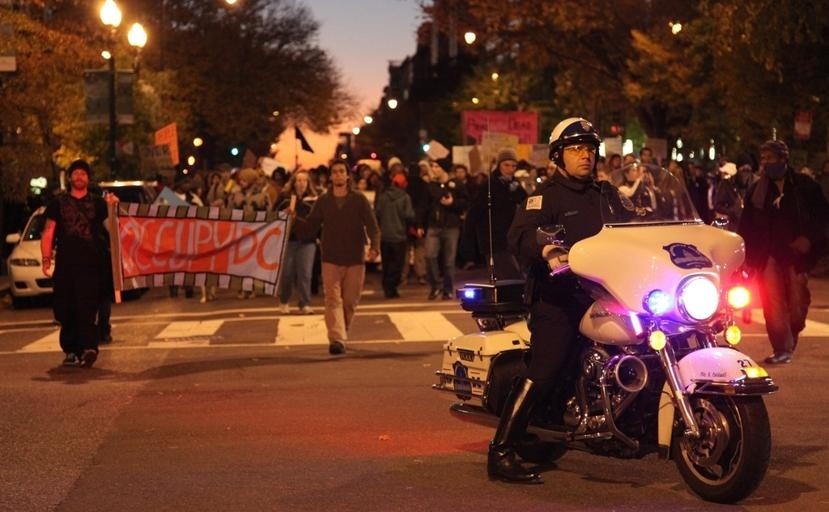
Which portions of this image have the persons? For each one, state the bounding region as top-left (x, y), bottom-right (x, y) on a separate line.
top-left (601, 138), bottom-right (828, 362)
top-left (146, 146), bottom-right (558, 316)
top-left (39, 160), bottom-right (120, 369)
top-left (298, 158), bottom-right (379, 356)
top-left (488, 118), bottom-right (665, 484)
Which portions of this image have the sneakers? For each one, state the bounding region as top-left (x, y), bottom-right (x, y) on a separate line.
top-left (328, 339), bottom-right (346, 357)
top-left (441, 288), bottom-right (453, 300)
top-left (278, 301), bottom-right (290, 315)
top-left (62, 352), bottom-right (76, 368)
top-left (75, 347), bottom-right (98, 370)
top-left (429, 286), bottom-right (440, 301)
top-left (297, 305), bottom-right (315, 315)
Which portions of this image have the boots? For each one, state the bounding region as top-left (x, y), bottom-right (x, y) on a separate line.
top-left (486, 373), bottom-right (545, 484)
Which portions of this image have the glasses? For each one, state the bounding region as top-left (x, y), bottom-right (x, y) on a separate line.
top-left (558, 143), bottom-right (599, 155)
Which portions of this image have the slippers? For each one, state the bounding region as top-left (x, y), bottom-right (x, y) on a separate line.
top-left (207, 294), bottom-right (218, 301)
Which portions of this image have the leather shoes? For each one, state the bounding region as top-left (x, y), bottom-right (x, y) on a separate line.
top-left (385, 288), bottom-right (402, 298)
top-left (760, 350), bottom-right (793, 364)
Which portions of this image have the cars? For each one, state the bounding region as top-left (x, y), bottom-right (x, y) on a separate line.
top-left (2, 207), bottom-right (55, 307)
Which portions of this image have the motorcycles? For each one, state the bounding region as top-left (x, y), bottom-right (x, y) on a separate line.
top-left (433, 158), bottom-right (780, 506)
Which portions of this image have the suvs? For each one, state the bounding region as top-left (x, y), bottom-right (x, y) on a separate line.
top-left (96, 180), bottom-right (159, 206)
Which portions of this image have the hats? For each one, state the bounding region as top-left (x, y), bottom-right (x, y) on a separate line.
top-left (393, 173), bottom-right (407, 189)
top-left (240, 168), bottom-right (260, 185)
top-left (496, 148), bottom-right (518, 166)
top-left (388, 156), bottom-right (401, 170)
top-left (719, 162), bottom-right (739, 176)
top-left (759, 139), bottom-right (790, 162)
top-left (418, 158), bottom-right (453, 172)
top-left (66, 160), bottom-right (93, 179)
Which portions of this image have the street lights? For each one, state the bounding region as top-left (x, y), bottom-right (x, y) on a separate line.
top-left (98, 0), bottom-right (148, 181)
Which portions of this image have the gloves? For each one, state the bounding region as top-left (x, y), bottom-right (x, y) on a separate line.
top-left (541, 243), bottom-right (574, 272)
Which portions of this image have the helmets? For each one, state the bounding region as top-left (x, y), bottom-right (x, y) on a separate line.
top-left (547, 117), bottom-right (602, 162)
